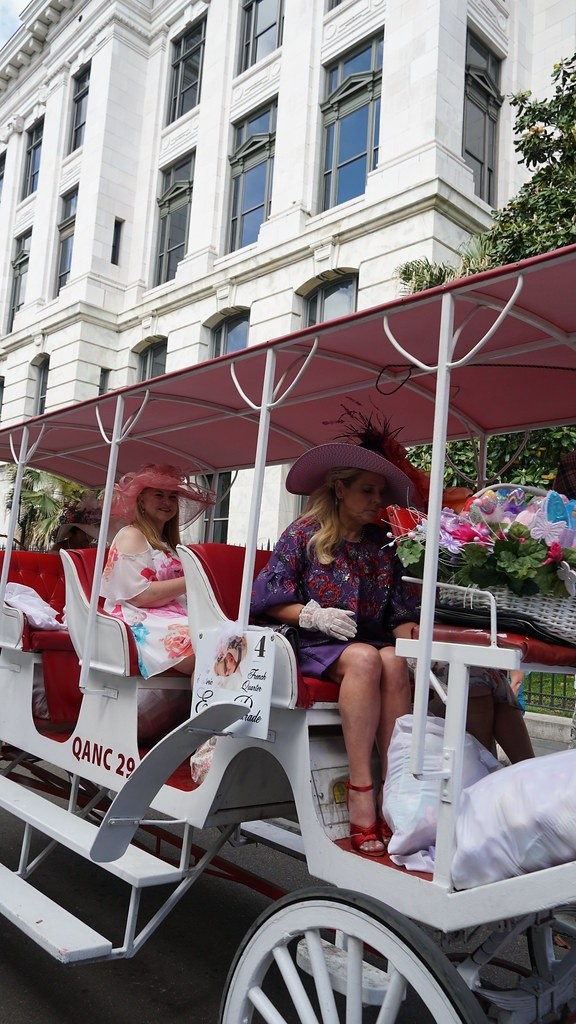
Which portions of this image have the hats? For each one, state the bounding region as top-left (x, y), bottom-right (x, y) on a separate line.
top-left (97, 463), bottom-right (214, 534)
top-left (286, 433), bottom-right (423, 506)
top-left (57, 494), bottom-right (100, 542)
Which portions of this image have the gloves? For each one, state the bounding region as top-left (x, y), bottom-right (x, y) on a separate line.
top-left (299, 599), bottom-right (358, 642)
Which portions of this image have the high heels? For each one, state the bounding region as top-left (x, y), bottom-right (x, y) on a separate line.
top-left (346, 777), bottom-right (393, 858)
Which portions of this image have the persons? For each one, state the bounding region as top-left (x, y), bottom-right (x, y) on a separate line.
top-left (52, 523), bottom-right (102, 551)
top-left (213, 635), bottom-right (247, 690)
top-left (251, 428), bottom-right (418, 858)
top-left (403, 655), bottom-right (535, 765)
top-left (98, 462), bottom-right (216, 688)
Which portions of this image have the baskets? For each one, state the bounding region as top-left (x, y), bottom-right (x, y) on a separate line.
top-left (440, 483), bottom-right (576, 643)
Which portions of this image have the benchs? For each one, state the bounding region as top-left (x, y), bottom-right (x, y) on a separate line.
top-left (387, 504), bottom-right (576, 675)
top-left (176, 543), bottom-right (339, 711)
top-left (57, 547), bottom-right (138, 679)
top-left (0, 550), bottom-right (74, 653)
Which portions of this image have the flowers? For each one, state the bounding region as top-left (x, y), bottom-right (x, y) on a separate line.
top-left (381, 483), bottom-right (576, 597)
top-left (49, 495), bottom-right (102, 544)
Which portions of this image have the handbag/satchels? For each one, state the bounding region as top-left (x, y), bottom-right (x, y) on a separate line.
top-left (382, 710), bottom-right (499, 871)
